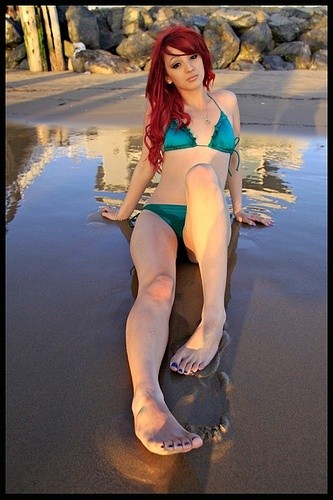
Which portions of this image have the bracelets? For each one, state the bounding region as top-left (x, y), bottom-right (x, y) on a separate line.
top-left (114, 211), bottom-right (129, 221)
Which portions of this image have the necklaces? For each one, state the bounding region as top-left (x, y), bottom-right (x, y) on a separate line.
top-left (183, 97), bottom-right (209, 126)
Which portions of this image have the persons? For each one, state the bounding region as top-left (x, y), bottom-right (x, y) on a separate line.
top-left (101, 26), bottom-right (270, 455)
